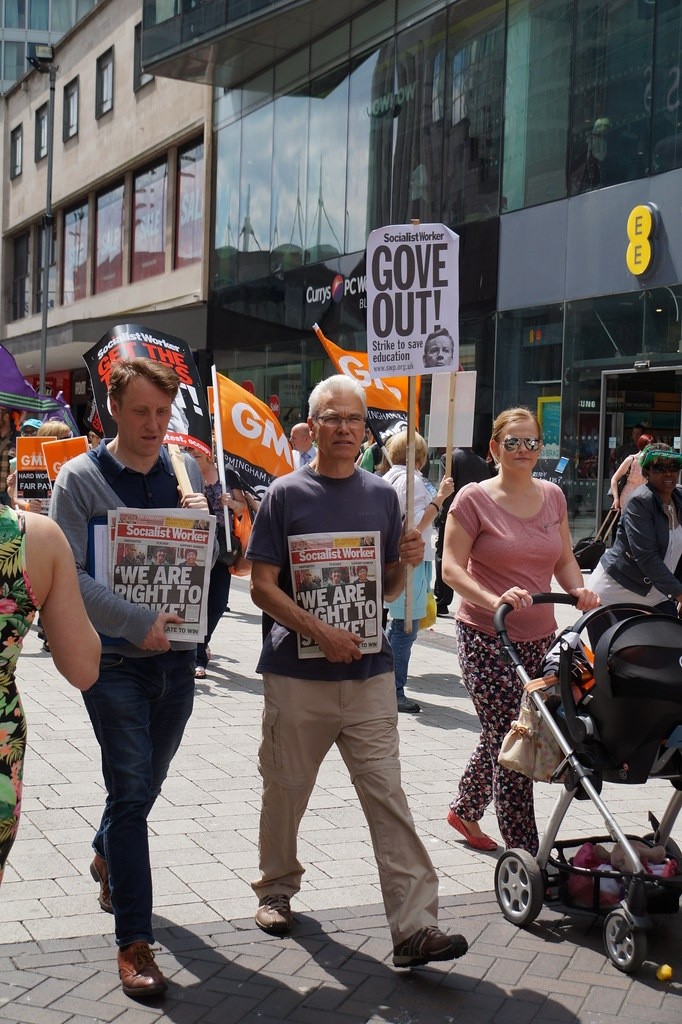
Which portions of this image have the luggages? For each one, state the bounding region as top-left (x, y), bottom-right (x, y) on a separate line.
top-left (572, 506), bottom-right (622, 573)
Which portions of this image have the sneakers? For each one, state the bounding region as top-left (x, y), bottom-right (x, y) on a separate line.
top-left (396, 695), bottom-right (420, 713)
top-left (392, 925), bottom-right (469, 967)
top-left (255, 893), bottom-right (293, 933)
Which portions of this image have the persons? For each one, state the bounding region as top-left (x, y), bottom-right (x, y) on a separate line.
top-left (422, 328), bottom-right (454, 368)
top-left (246, 375), bottom-right (467, 966)
top-left (48, 358), bottom-right (230, 994)
top-left (442, 410), bottom-right (601, 896)
top-left (289, 423), bottom-right (318, 469)
top-left (410, 144), bottom-right (501, 223)
top-left (573, 425), bottom-right (682, 653)
top-left (1, 419), bottom-right (102, 880)
top-left (185, 445), bottom-right (247, 677)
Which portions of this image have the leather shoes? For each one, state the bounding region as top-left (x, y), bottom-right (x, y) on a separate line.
top-left (117, 939), bottom-right (167, 997)
top-left (89, 854), bottom-right (114, 914)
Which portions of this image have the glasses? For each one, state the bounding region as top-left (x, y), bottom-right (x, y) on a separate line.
top-left (652, 461), bottom-right (682, 472)
top-left (500, 434), bottom-right (543, 455)
top-left (312, 413), bottom-right (367, 428)
top-left (21, 430), bottom-right (38, 436)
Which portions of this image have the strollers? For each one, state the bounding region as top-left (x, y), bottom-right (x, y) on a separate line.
top-left (494, 592), bottom-right (682, 973)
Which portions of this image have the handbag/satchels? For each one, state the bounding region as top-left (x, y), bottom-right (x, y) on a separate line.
top-left (497, 673), bottom-right (571, 784)
top-left (607, 456), bottom-right (634, 500)
top-left (418, 591), bottom-right (437, 630)
top-left (227, 501), bottom-right (254, 576)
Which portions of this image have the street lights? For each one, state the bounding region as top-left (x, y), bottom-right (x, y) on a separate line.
top-left (26, 43), bottom-right (59, 428)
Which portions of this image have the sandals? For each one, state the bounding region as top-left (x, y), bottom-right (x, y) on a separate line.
top-left (204, 644), bottom-right (211, 661)
top-left (193, 665), bottom-right (206, 678)
top-left (447, 810), bottom-right (498, 850)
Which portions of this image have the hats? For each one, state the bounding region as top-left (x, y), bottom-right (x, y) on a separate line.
top-left (19, 418), bottom-right (43, 432)
top-left (638, 442), bottom-right (682, 475)
top-left (185, 549), bottom-right (197, 558)
top-left (358, 566), bottom-right (368, 573)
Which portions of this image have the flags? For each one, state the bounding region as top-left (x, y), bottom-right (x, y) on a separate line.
top-left (313, 324), bottom-right (421, 446)
top-left (207, 365), bottom-right (294, 524)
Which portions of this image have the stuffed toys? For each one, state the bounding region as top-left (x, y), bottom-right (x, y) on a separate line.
top-left (185, 22), bottom-right (205, 42)
top-left (593, 841), bottom-right (666, 871)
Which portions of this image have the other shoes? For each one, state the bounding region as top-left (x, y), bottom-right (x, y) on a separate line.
top-left (437, 604), bottom-right (449, 614)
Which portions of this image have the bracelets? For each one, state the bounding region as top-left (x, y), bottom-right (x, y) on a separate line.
top-left (429, 502), bottom-right (441, 516)
